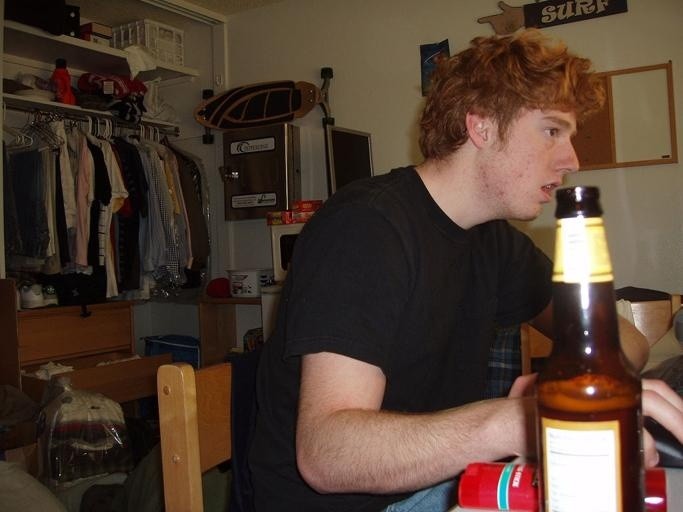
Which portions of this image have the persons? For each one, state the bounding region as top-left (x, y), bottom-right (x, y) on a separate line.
top-left (241, 26), bottom-right (682, 512)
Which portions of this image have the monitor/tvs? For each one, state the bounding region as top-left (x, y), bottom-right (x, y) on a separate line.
top-left (324, 125), bottom-right (374, 199)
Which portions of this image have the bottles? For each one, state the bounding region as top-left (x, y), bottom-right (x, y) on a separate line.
top-left (537, 185), bottom-right (648, 510)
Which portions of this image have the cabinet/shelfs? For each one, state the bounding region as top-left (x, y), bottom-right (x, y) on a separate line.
top-left (0, 277), bottom-right (181, 418)
top-left (0, 19), bottom-right (202, 127)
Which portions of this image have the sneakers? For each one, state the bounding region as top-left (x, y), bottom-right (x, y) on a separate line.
top-left (16, 282), bottom-right (58, 312)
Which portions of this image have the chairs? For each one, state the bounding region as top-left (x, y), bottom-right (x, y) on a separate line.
top-left (521, 293), bottom-right (683, 368)
top-left (156, 357), bottom-right (236, 512)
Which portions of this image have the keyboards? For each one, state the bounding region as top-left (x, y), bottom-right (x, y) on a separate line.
top-left (640, 352), bottom-right (683, 396)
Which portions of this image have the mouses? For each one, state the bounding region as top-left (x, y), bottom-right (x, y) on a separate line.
top-left (643, 417), bottom-right (683, 469)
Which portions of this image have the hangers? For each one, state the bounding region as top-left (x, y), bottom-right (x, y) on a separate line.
top-left (1, 102), bottom-right (183, 153)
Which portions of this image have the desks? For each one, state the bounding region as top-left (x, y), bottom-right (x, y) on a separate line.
top-left (196, 295), bottom-right (263, 369)
